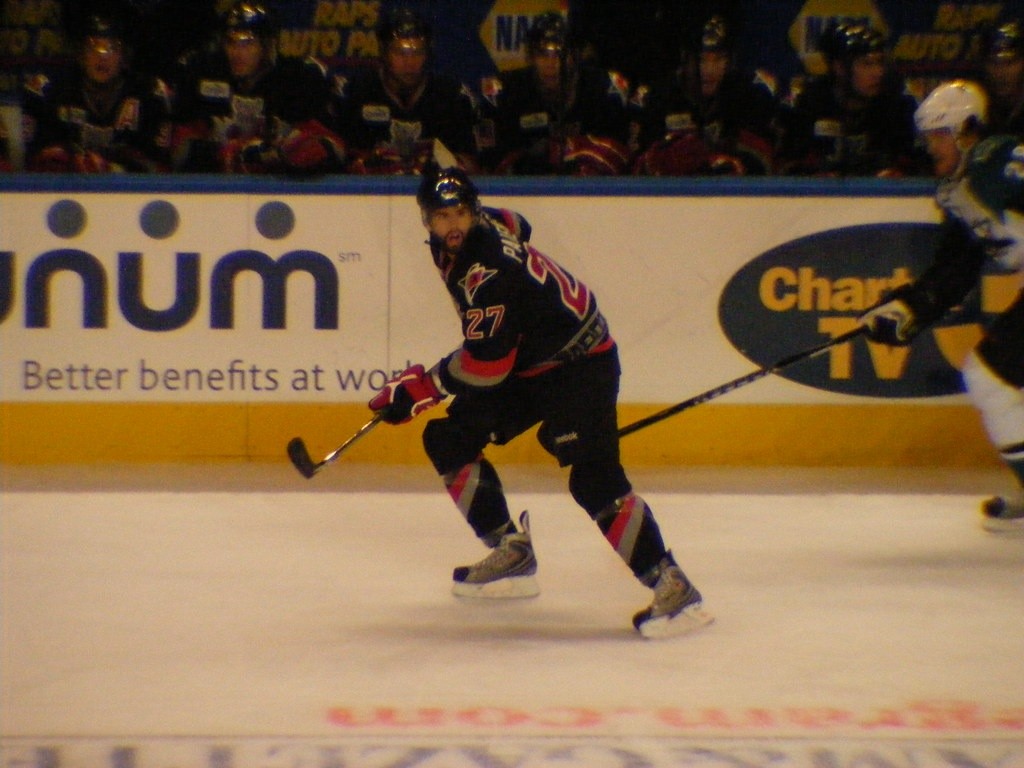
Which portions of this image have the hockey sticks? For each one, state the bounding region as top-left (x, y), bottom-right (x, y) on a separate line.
top-left (285, 357), bottom-right (444, 478)
top-left (618, 325), bottom-right (870, 437)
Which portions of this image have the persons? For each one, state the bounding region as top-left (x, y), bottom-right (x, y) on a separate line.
top-left (859, 81), bottom-right (1024, 530)
top-left (0, 0), bottom-right (1024, 176)
top-left (368, 167), bottom-right (715, 640)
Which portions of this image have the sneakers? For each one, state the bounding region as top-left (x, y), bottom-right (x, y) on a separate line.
top-left (451, 511), bottom-right (539, 599)
top-left (981, 494), bottom-right (1024, 536)
top-left (633, 548), bottom-right (714, 640)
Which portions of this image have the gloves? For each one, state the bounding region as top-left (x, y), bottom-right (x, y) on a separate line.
top-left (369, 364), bottom-right (448, 425)
top-left (856, 283), bottom-right (944, 347)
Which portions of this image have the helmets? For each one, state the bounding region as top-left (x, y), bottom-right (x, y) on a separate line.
top-left (381, 6), bottom-right (426, 53)
top-left (525, 12), bottom-right (575, 58)
top-left (225, 2), bottom-right (276, 47)
top-left (698, 15), bottom-right (731, 54)
top-left (78, 13), bottom-right (125, 52)
top-left (819, 21), bottom-right (884, 69)
top-left (912, 80), bottom-right (988, 136)
top-left (418, 167), bottom-right (479, 217)
top-left (984, 19), bottom-right (1024, 63)
